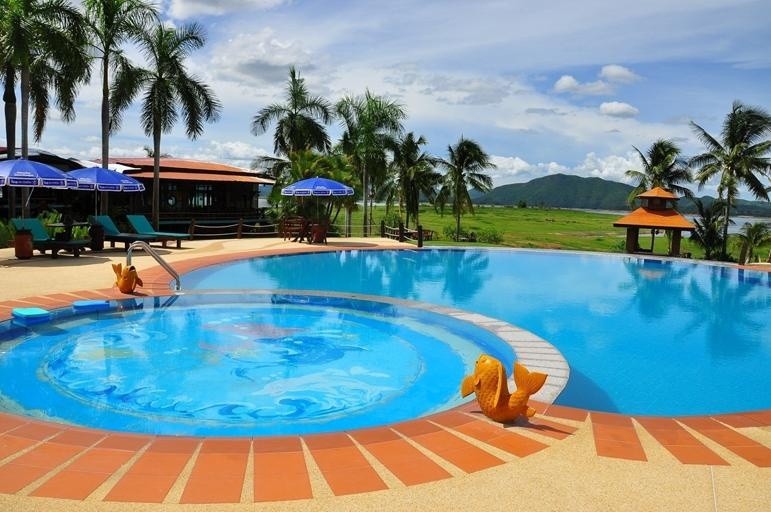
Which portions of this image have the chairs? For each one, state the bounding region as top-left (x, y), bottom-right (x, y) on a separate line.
top-left (284, 214), bottom-right (332, 245)
top-left (8, 214), bottom-right (192, 258)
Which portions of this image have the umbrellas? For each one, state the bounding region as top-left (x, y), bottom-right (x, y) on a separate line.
top-left (281, 176), bottom-right (354, 221)
top-left (1, 158), bottom-right (77, 217)
top-left (64, 166), bottom-right (147, 219)
